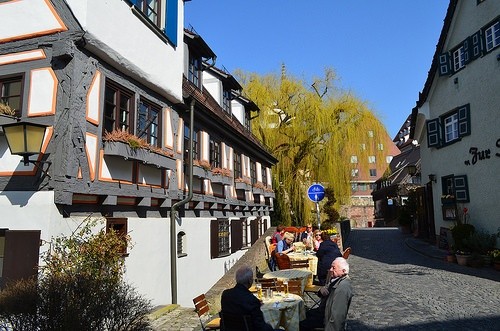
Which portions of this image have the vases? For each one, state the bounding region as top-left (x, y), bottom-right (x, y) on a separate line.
top-left (464, 251), bottom-right (476, 258)
top-left (495, 261), bottom-right (500, 271)
top-left (446, 231), bottom-right (464, 248)
top-left (471, 259), bottom-right (482, 267)
top-left (479, 255), bottom-right (491, 265)
top-left (454, 254), bottom-right (472, 266)
top-left (447, 255), bottom-right (454, 262)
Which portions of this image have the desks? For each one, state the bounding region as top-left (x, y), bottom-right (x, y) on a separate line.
top-left (286, 252), bottom-right (318, 275)
top-left (262, 269), bottom-right (312, 297)
top-left (253, 291), bottom-right (307, 331)
top-left (292, 241), bottom-right (313, 252)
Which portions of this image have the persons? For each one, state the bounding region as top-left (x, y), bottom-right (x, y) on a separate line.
top-left (271, 224), bottom-right (343, 286)
top-left (300, 256), bottom-right (354, 331)
top-left (220, 267), bottom-right (285, 331)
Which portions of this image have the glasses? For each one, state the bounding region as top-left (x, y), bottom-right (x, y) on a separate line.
top-left (288, 238), bottom-right (293, 243)
top-left (315, 234), bottom-right (321, 236)
top-left (307, 227), bottom-right (311, 229)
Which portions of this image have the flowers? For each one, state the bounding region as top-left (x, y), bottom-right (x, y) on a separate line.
top-left (445, 206), bottom-right (500, 262)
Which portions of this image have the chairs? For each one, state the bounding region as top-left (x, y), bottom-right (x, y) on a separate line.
top-left (255, 236), bottom-right (354, 331)
top-left (192, 293), bottom-right (222, 331)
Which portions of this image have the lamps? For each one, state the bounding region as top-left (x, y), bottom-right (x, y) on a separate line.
top-left (0, 120), bottom-right (52, 179)
top-left (407, 163), bottom-right (420, 178)
top-left (428, 174), bottom-right (437, 183)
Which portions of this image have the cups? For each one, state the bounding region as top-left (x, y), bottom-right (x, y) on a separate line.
top-left (256, 277), bottom-right (289, 298)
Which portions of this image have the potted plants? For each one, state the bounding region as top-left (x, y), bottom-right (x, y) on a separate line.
top-left (102, 128), bottom-right (177, 173)
top-left (185, 159), bottom-right (234, 186)
top-left (0, 102), bottom-right (20, 134)
top-left (234, 177), bottom-right (275, 198)
top-left (398, 207), bottom-right (411, 234)
